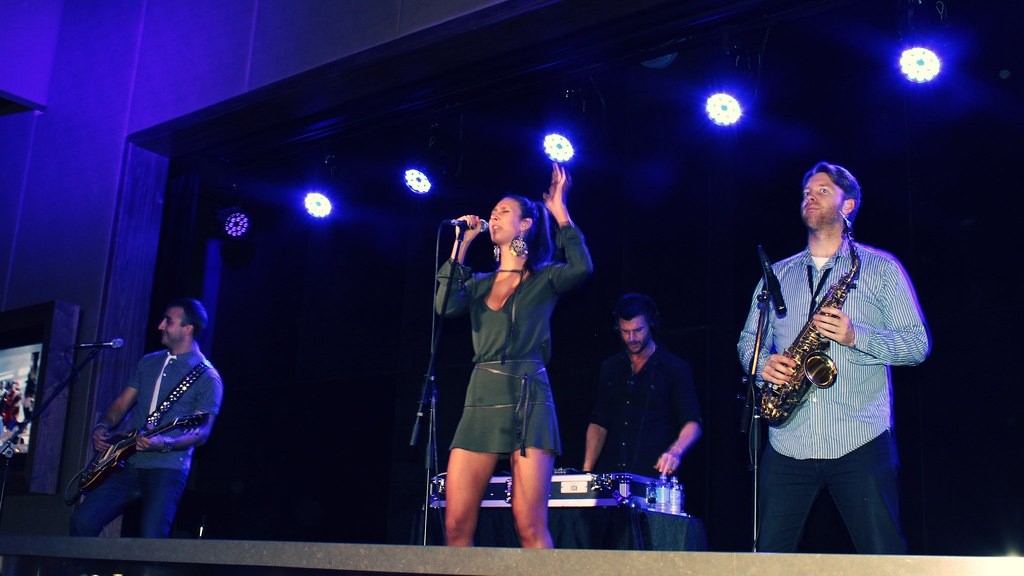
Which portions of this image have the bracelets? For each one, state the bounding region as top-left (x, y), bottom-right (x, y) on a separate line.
top-left (161, 437), bottom-right (175, 453)
top-left (95, 423), bottom-right (105, 427)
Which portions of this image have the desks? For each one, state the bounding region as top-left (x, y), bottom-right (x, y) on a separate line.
top-left (407, 505), bottom-right (709, 552)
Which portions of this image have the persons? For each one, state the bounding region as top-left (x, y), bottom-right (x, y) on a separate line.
top-left (67, 298), bottom-right (224, 575)
top-left (433, 163), bottom-right (593, 548)
top-left (582, 294), bottom-right (703, 551)
top-left (0, 380), bottom-right (34, 444)
top-left (736, 162), bottom-right (931, 557)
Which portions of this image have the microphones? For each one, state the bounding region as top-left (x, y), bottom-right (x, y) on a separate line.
top-left (757, 244), bottom-right (787, 314)
top-left (443, 219), bottom-right (489, 232)
top-left (74, 338), bottom-right (124, 349)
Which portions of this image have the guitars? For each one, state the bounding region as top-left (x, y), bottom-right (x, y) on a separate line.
top-left (80, 411), bottom-right (211, 492)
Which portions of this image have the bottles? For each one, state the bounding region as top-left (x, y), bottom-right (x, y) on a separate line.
top-left (655, 472), bottom-right (669, 513)
top-left (669, 476), bottom-right (682, 515)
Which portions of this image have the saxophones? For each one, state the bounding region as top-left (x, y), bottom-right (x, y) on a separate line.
top-left (761, 218), bottom-right (862, 428)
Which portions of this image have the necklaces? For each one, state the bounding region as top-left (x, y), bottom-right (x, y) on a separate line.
top-left (497, 270), bottom-right (528, 341)
